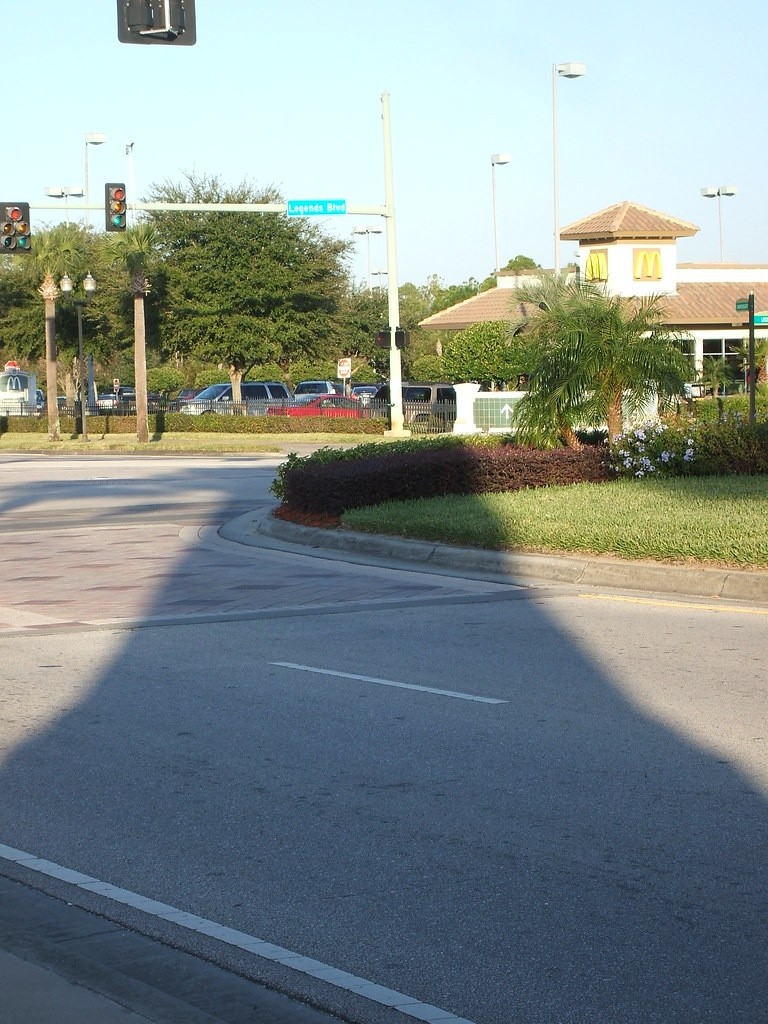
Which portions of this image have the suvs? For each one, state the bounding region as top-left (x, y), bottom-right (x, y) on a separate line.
top-left (292, 380), bottom-right (336, 400)
top-left (179, 378), bottom-right (294, 416)
top-left (368, 381), bottom-right (456, 422)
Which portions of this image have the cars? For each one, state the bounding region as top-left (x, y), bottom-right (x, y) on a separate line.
top-left (350, 385), bottom-right (378, 405)
top-left (266, 392), bottom-right (371, 419)
top-left (33, 385), bottom-right (211, 414)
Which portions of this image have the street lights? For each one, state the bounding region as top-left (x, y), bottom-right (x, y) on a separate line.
top-left (547, 61), bottom-right (585, 290)
top-left (700, 181), bottom-right (736, 260)
top-left (80, 130), bottom-right (109, 235)
top-left (44, 184), bottom-right (84, 231)
top-left (58, 269), bottom-right (100, 442)
top-left (370, 269), bottom-right (389, 296)
top-left (489, 150), bottom-right (510, 271)
top-left (351, 226), bottom-right (383, 301)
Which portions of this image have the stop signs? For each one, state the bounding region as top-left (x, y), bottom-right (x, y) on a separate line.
top-left (5, 360), bottom-right (20, 373)
top-left (113, 378), bottom-right (121, 393)
top-left (336, 357), bottom-right (352, 379)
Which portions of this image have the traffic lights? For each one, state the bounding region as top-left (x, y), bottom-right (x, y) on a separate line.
top-left (395, 329), bottom-right (410, 348)
top-left (375, 331), bottom-right (392, 350)
top-left (0, 200), bottom-right (32, 255)
top-left (103, 181), bottom-right (128, 232)
